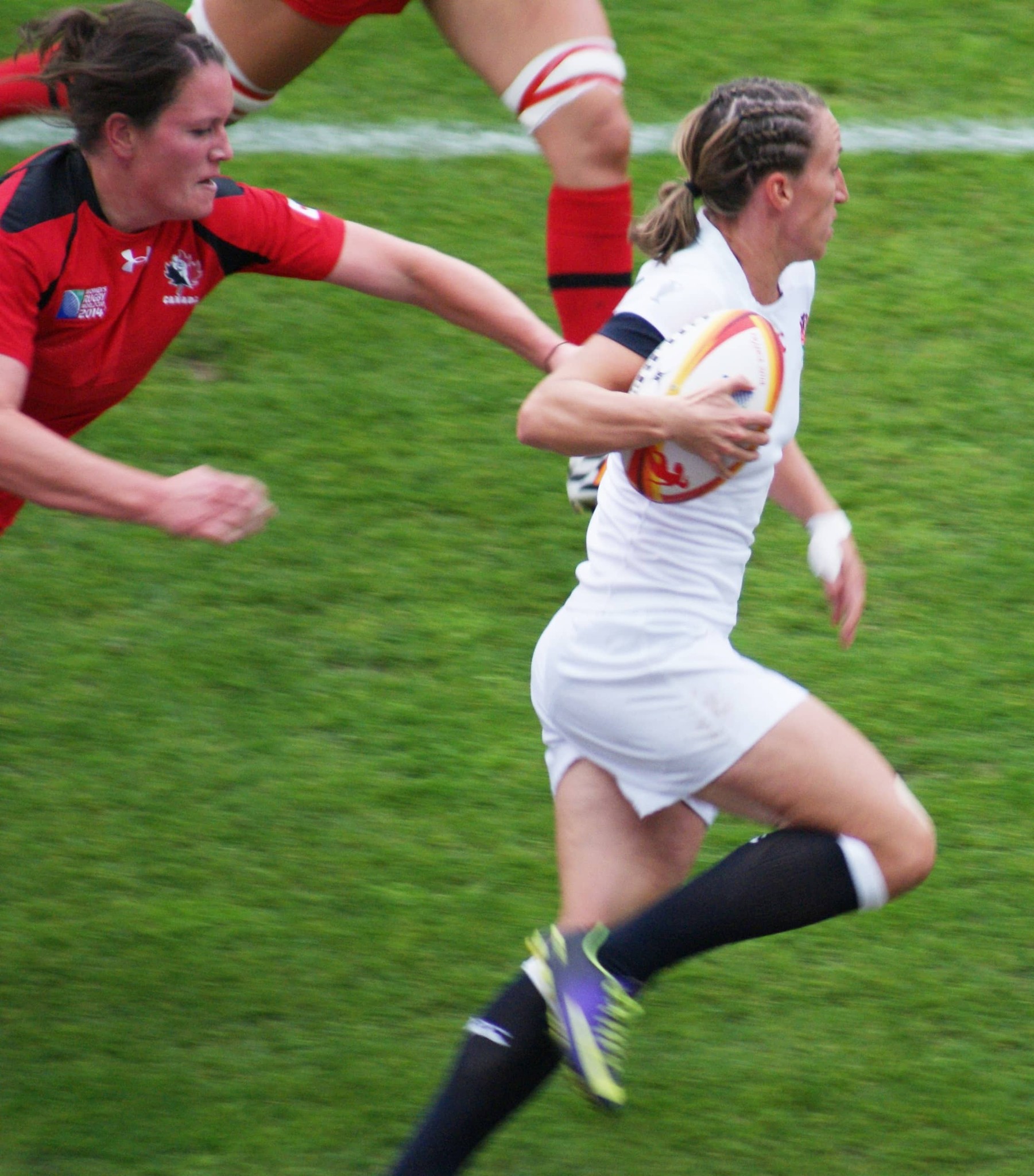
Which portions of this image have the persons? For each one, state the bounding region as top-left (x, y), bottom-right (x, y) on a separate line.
top-left (0, 10), bottom-right (584, 544)
top-left (389, 76), bottom-right (936, 1176)
top-left (0, 0), bottom-right (636, 512)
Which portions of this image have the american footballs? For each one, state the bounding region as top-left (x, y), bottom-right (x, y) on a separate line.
top-left (616, 306), bottom-right (783, 505)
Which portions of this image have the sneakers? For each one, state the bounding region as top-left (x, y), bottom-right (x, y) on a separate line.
top-left (521, 920), bottom-right (647, 1114)
top-left (566, 450), bottom-right (608, 513)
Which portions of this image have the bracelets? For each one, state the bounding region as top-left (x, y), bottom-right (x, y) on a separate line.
top-left (542, 340), bottom-right (572, 369)
